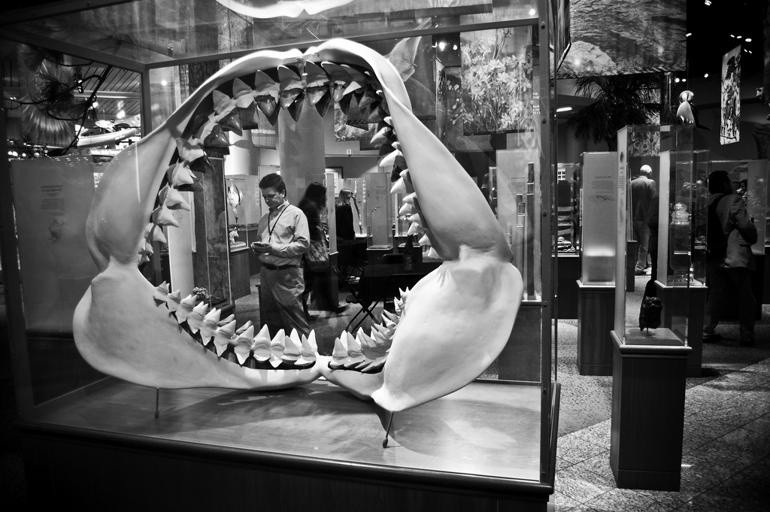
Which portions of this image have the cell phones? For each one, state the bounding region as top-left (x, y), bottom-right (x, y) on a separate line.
top-left (254, 242), bottom-right (261, 246)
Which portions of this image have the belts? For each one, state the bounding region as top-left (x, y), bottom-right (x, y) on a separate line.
top-left (261, 260), bottom-right (298, 272)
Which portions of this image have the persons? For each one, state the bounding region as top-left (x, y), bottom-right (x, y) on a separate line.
top-left (249, 173), bottom-right (311, 342)
top-left (631, 164), bottom-right (657, 274)
top-left (703, 171), bottom-right (762, 346)
top-left (739, 179), bottom-right (748, 204)
top-left (298, 181), bottom-right (350, 321)
top-left (335, 188), bottom-right (363, 277)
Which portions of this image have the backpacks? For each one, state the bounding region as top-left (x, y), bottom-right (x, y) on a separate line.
top-left (690, 191), bottom-right (740, 269)
